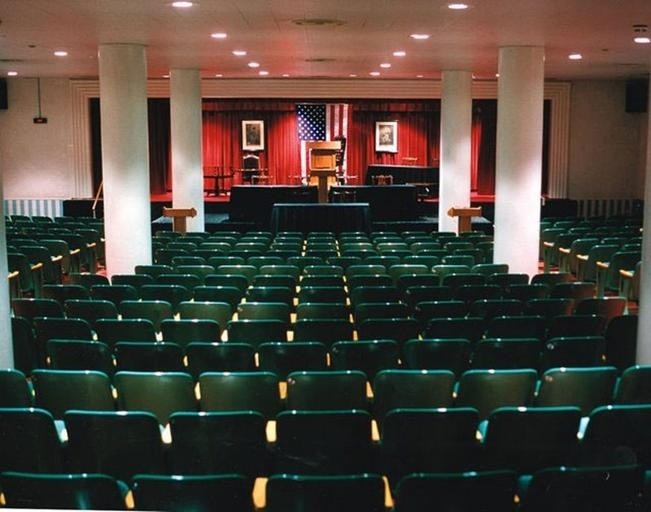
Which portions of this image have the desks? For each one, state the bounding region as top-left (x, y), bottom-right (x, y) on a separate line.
top-left (228, 163), bottom-right (439, 232)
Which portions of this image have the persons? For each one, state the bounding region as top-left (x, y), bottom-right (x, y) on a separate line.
top-left (247, 127), bottom-right (259, 143)
top-left (374, 173), bottom-right (386, 185)
top-left (381, 125), bottom-right (391, 143)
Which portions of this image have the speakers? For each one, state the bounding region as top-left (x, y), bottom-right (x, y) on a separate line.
top-left (0, 78), bottom-right (8, 109)
top-left (624, 79), bottom-right (648, 112)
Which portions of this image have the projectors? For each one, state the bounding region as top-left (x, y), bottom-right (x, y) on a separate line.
top-left (32, 117), bottom-right (47, 124)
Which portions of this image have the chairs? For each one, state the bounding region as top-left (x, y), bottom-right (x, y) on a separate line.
top-left (401, 156), bottom-right (418, 166)
top-left (241, 154), bottom-right (261, 185)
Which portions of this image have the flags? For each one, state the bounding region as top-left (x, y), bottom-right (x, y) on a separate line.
top-left (294, 103), bottom-right (350, 141)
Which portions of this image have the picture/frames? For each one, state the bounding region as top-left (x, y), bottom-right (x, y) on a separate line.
top-left (240, 119), bottom-right (267, 153)
top-left (374, 120), bottom-right (399, 154)
